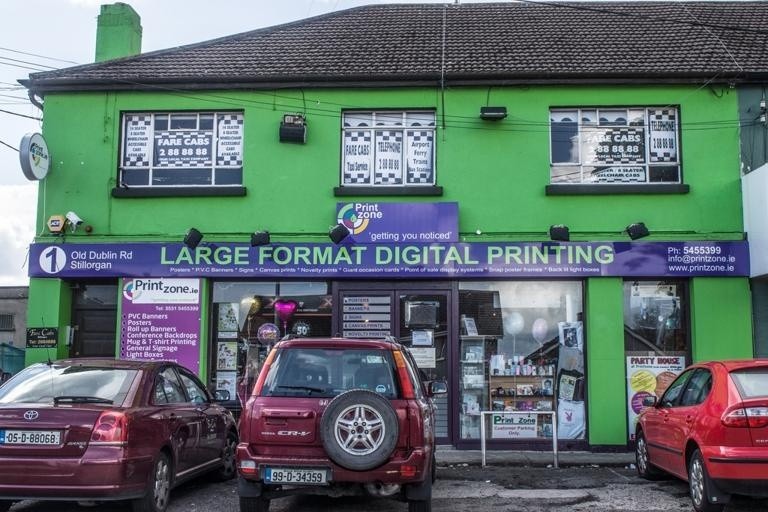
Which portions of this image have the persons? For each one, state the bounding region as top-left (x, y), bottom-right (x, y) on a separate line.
top-left (542, 379), bottom-right (552, 395)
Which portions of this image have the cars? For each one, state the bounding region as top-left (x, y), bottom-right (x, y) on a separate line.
top-left (636, 358), bottom-right (768, 512)
top-left (0, 316), bottom-right (239, 512)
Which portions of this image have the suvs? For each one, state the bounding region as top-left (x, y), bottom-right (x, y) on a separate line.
top-left (236, 334), bottom-right (449, 512)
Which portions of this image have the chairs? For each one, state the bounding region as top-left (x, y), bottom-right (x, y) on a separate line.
top-left (289, 363), bottom-right (328, 389)
top-left (354, 368), bottom-right (392, 395)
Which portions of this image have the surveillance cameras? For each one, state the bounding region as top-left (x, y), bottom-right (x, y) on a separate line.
top-left (65, 212), bottom-right (84, 226)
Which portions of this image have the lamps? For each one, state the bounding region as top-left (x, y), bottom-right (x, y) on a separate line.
top-left (550, 222), bottom-right (570, 242)
top-left (184, 227), bottom-right (203, 251)
top-left (626, 220), bottom-right (650, 241)
top-left (327, 222), bottom-right (350, 245)
top-left (248, 230), bottom-right (273, 246)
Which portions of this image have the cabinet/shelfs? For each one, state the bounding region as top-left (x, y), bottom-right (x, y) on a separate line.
top-left (459, 335), bottom-right (497, 438)
top-left (488, 363), bottom-right (556, 439)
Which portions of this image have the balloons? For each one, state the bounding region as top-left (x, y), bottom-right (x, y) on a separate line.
top-left (256, 323), bottom-right (279, 352)
top-left (239, 296), bottom-right (261, 321)
top-left (273, 298), bottom-right (296, 326)
top-left (531, 318), bottom-right (549, 347)
top-left (503, 312), bottom-right (525, 336)
